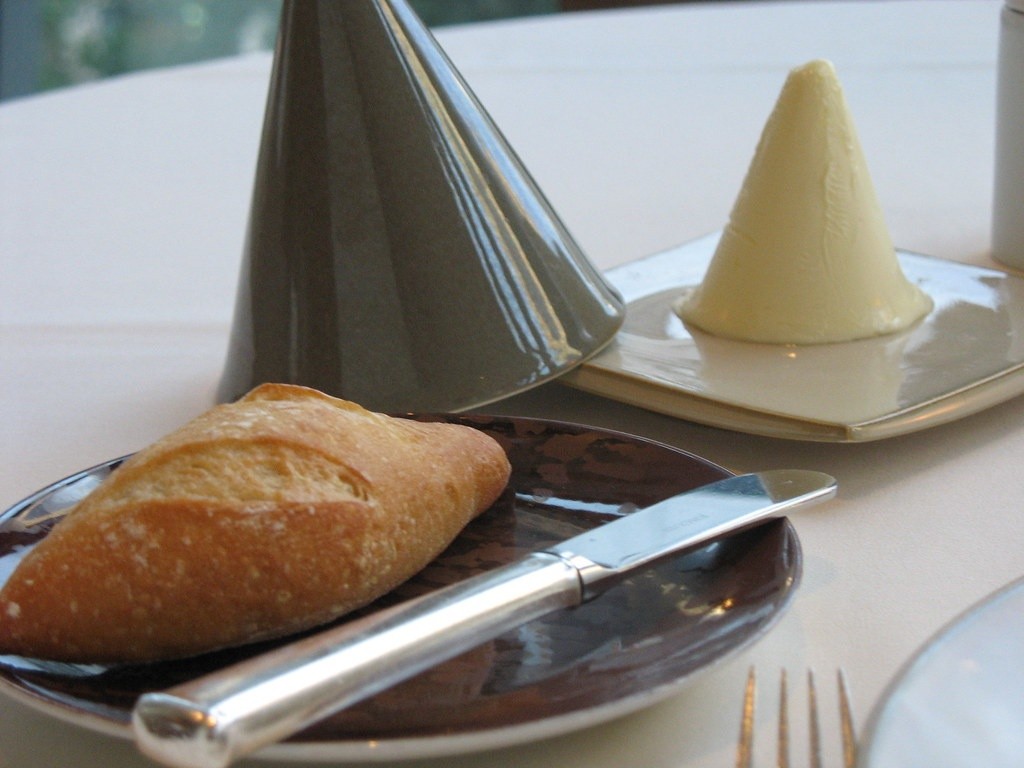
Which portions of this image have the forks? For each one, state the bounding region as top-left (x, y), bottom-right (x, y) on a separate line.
top-left (737, 663), bottom-right (857, 768)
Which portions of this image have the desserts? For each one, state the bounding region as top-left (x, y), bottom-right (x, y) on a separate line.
top-left (672, 60), bottom-right (934, 344)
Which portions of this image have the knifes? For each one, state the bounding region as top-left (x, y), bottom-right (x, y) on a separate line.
top-left (130, 469), bottom-right (837, 768)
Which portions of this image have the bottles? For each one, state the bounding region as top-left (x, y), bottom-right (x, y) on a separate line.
top-left (991, 0), bottom-right (1024, 269)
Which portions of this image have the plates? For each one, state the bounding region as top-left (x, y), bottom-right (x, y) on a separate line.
top-left (0, 413), bottom-right (806, 763)
top-left (558, 228), bottom-right (1024, 446)
top-left (854, 576), bottom-right (1024, 768)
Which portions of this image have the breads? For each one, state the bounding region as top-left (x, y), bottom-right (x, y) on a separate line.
top-left (0, 383), bottom-right (512, 665)
